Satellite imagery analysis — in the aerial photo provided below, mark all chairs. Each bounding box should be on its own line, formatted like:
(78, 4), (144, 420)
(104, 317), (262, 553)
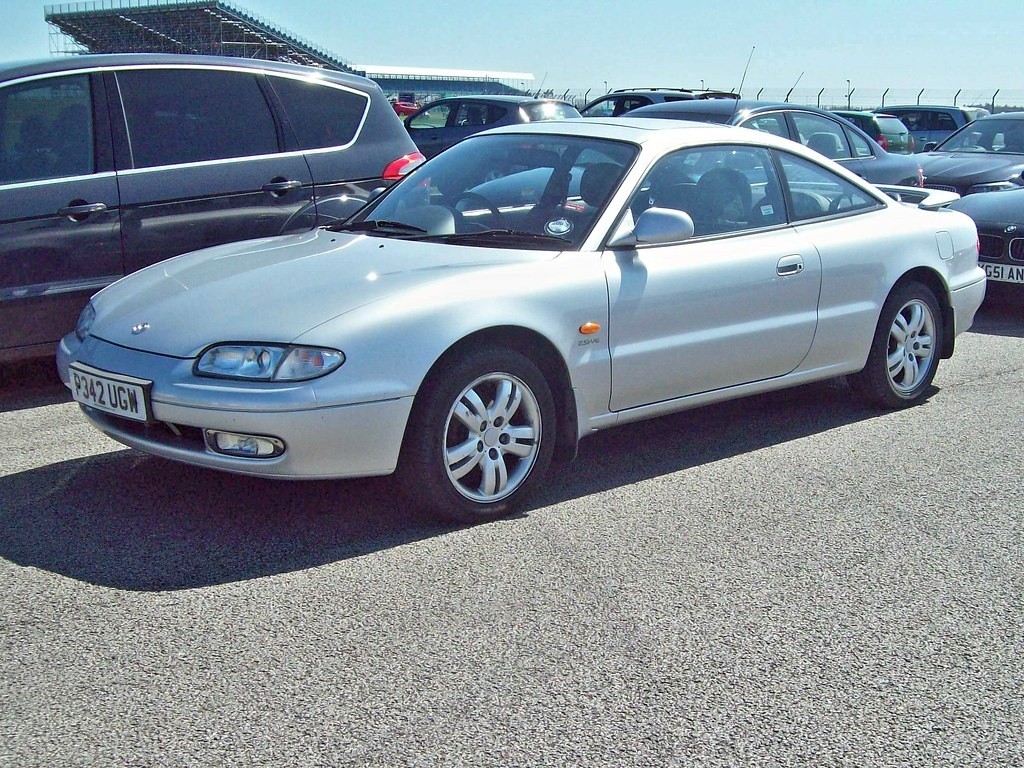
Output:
(997, 125), (1024, 154)
(806, 132), (847, 160)
(518, 162), (833, 257)
(461, 107), (486, 127)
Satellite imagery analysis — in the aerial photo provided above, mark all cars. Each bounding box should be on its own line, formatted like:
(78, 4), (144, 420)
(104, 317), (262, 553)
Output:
(911, 110), (1024, 207)
(455, 100), (925, 241)
(53, 116), (986, 524)
(944, 173), (1024, 302)
(762, 110), (917, 158)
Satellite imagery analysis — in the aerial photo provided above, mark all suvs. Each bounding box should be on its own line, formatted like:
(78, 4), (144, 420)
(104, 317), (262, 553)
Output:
(404, 95), (587, 195)
(394, 102), (429, 119)
(0, 54), (429, 367)
(870, 105), (993, 150)
(577, 87), (758, 130)
